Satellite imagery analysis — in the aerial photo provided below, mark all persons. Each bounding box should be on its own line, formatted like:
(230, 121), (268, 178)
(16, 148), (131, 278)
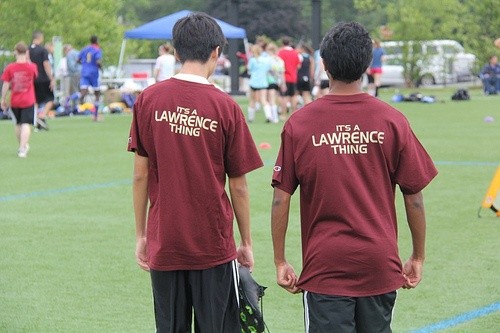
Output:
(127, 15), (265, 333)
(269, 21), (438, 333)
(0, 10), (388, 160)
(480, 55), (500, 94)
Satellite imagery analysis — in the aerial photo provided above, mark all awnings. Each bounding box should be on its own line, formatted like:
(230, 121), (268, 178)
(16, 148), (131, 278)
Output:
(126, 10), (245, 39)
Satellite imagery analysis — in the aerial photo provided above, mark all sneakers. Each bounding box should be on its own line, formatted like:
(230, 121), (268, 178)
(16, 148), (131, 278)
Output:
(238, 262), (270, 333)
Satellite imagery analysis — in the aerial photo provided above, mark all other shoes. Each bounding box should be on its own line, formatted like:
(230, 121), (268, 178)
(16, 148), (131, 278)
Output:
(34, 117), (48, 131)
(18, 143), (29, 156)
(80, 95), (84, 104)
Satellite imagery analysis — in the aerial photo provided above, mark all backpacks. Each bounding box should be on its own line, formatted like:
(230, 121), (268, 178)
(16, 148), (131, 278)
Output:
(452, 88), (471, 99)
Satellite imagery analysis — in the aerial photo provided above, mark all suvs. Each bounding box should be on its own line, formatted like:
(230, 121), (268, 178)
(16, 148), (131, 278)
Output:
(381, 40), (480, 88)
